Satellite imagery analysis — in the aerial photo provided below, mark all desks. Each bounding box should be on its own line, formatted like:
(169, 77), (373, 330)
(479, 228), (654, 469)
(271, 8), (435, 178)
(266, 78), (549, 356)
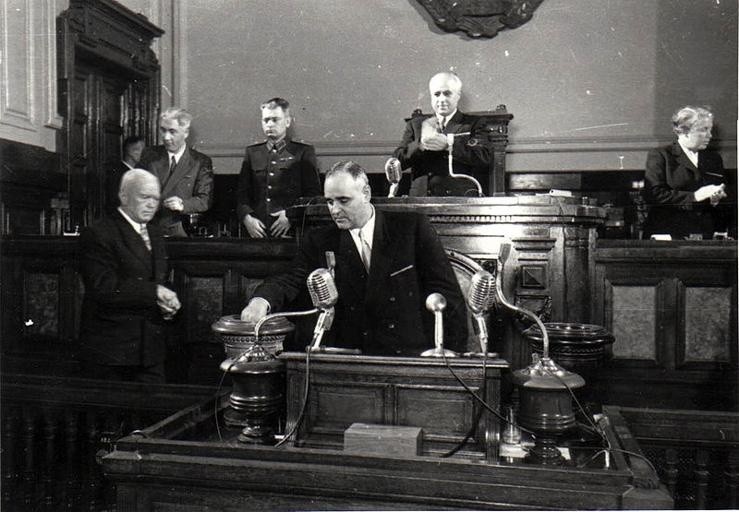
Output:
(2, 198), (735, 409)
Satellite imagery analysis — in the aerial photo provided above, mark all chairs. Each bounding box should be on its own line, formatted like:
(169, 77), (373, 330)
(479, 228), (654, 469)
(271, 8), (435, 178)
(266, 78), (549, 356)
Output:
(404, 102), (515, 195)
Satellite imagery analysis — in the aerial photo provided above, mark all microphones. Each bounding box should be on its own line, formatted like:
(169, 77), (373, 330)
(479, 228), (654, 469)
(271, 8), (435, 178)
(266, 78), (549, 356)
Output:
(425, 293), (447, 317)
(307, 267), (340, 311)
(385, 158), (403, 184)
(467, 272), (496, 314)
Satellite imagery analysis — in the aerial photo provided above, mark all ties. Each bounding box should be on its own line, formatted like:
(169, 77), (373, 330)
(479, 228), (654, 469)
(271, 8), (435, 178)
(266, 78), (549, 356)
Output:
(359, 231), (371, 270)
(170, 156), (177, 172)
(441, 115), (447, 131)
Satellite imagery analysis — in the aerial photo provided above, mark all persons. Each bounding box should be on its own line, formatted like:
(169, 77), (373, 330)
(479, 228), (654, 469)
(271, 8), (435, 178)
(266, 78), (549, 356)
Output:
(391, 70), (495, 195)
(72, 166), (183, 381)
(238, 159), (468, 356)
(235, 97), (323, 239)
(639, 105), (732, 240)
(133, 107), (215, 237)
(105, 135), (146, 216)
